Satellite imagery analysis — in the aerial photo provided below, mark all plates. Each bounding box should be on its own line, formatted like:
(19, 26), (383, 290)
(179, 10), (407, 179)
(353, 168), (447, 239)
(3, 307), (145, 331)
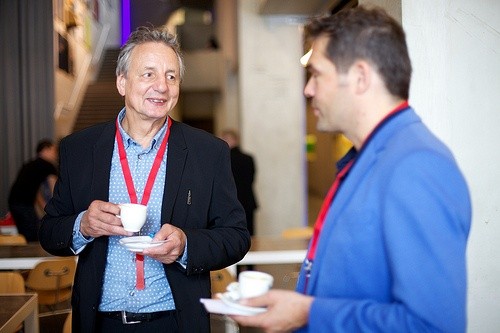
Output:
(222, 293), (266, 312)
(120, 240), (166, 249)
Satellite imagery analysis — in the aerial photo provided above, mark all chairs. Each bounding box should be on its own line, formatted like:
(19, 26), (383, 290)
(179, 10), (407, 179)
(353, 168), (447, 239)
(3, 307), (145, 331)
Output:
(25, 259), (76, 333)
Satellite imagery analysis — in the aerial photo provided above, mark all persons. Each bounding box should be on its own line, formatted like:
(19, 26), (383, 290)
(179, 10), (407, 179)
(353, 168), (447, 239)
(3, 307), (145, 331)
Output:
(38, 22), (252, 333)
(222, 130), (259, 236)
(8, 139), (56, 244)
(228, 7), (472, 333)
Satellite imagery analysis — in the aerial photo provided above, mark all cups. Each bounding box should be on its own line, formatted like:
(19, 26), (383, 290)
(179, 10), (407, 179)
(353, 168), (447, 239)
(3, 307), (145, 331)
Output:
(116, 204), (147, 232)
(239, 271), (273, 296)
